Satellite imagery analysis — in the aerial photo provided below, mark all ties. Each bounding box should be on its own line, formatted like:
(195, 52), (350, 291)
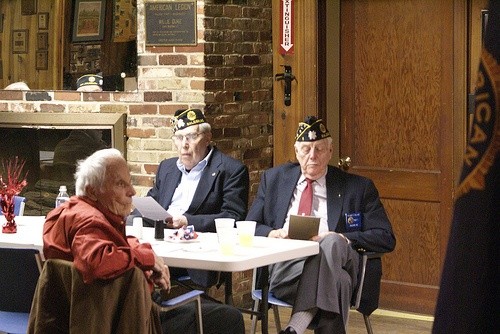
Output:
(298, 178), (316, 216)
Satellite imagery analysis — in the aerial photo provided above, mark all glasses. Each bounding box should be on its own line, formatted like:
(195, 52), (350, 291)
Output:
(172, 130), (207, 142)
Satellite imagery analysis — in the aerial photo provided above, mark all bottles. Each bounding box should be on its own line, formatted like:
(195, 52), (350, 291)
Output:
(55, 186), (69, 208)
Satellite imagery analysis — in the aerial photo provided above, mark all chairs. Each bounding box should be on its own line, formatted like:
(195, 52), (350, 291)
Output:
(0, 247), (46, 334)
(251, 251), (383, 333)
(27, 259), (204, 333)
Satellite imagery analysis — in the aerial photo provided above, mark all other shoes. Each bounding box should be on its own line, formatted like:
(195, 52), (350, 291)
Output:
(279, 326), (297, 334)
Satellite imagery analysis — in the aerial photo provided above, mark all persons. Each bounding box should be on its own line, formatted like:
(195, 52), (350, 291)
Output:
(42, 148), (247, 334)
(123, 109), (250, 287)
(246, 116), (396, 334)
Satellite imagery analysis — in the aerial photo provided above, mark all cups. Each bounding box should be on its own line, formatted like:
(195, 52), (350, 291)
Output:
(155, 220), (164, 239)
(236, 221), (257, 242)
(214, 218), (236, 241)
(133, 217), (143, 238)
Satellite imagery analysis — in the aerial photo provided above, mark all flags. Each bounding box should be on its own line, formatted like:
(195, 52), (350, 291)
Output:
(432, 0), (500, 334)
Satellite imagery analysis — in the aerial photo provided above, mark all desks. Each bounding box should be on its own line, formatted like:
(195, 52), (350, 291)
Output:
(1, 212), (319, 334)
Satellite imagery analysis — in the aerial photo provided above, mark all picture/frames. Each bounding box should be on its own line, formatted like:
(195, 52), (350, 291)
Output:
(72, 0), (107, 43)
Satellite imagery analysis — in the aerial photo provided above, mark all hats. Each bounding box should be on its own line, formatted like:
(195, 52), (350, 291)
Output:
(77, 74), (103, 88)
(295, 115), (331, 142)
(170, 108), (207, 134)
